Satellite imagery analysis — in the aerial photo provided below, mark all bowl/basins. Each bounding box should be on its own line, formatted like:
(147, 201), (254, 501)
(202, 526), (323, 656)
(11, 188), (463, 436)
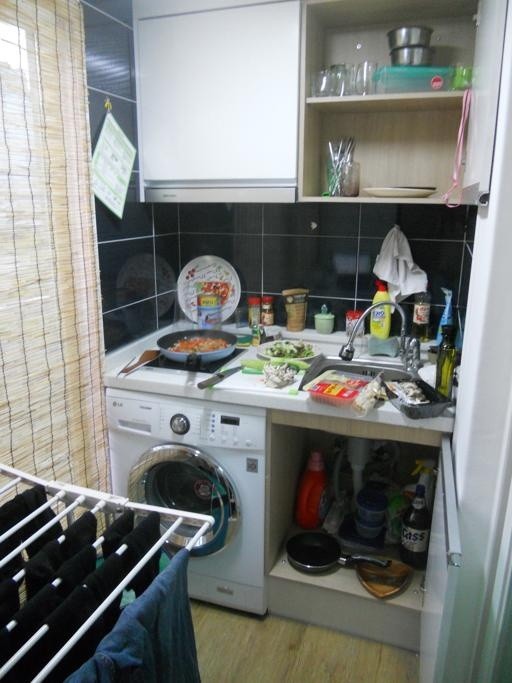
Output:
(386, 22), (435, 50)
(389, 45), (434, 67)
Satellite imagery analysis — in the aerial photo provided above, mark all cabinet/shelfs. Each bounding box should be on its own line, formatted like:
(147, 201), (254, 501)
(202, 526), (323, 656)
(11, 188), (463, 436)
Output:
(131, 1), (302, 190)
(296, 0), (506, 203)
(266, 409), (461, 682)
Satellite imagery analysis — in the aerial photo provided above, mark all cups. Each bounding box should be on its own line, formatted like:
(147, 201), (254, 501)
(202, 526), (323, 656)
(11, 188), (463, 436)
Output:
(313, 61), (379, 97)
(325, 153), (361, 197)
(314, 313), (335, 334)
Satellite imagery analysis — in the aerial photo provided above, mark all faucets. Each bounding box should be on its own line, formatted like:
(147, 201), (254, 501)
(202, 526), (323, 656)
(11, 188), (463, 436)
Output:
(339, 299), (408, 362)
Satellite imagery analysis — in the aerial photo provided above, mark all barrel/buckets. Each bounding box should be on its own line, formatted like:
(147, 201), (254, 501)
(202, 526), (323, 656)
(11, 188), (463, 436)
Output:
(352, 484), (387, 540)
(294, 451), (336, 529)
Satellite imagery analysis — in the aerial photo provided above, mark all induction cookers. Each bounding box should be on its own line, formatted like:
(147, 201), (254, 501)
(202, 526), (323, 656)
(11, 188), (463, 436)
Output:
(143, 346), (248, 374)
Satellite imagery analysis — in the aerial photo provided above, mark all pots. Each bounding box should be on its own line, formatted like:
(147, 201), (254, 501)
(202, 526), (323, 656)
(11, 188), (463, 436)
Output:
(285, 531), (388, 574)
(155, 327), (238, 369)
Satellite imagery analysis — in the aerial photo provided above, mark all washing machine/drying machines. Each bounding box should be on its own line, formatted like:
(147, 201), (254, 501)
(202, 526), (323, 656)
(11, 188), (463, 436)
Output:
(105, 388), (268, 618)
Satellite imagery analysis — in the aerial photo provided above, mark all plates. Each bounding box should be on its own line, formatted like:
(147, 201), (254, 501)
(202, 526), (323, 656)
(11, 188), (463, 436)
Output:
(256, 339), (323, 361)
(362, 187), (438, 198)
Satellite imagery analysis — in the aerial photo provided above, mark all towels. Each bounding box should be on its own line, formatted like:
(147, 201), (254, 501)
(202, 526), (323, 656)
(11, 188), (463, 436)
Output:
(372, 226), (428, 314)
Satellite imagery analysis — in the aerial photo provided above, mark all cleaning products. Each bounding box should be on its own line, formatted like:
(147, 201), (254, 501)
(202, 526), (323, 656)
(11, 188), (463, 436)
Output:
(369, 279), (392, 340)
(410, 458), (438, 515)
(385, 489), (410, 543)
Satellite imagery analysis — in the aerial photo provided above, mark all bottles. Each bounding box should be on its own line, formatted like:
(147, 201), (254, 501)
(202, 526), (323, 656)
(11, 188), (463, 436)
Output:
(399, 483), (432, 571)
(435, 324), (458, 400)
(248, 296), (261, 328)
(261, 295), (275, 326)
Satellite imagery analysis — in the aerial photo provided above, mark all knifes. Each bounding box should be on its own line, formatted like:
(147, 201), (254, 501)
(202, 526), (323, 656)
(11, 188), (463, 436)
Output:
(198, 366), (242, 389)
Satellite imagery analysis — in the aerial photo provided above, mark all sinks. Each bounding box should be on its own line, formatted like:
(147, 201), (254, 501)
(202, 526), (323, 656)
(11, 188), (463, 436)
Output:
(297, 352), (422, 393)
